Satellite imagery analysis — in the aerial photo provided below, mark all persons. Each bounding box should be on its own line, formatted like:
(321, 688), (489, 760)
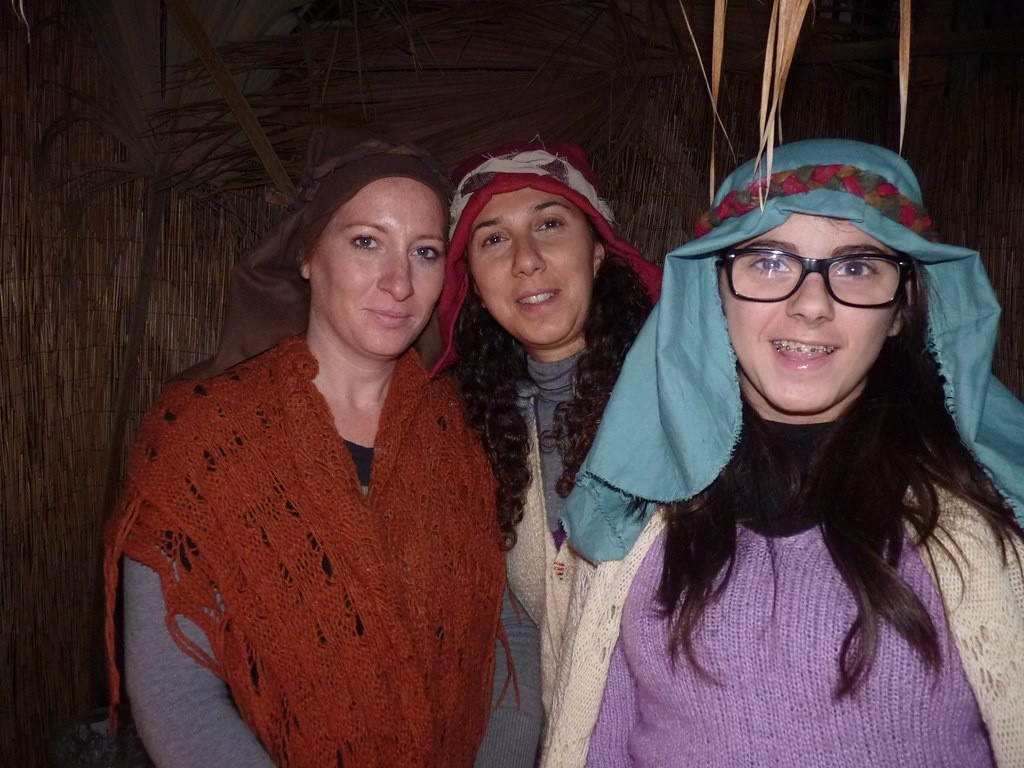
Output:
(535, 141), (1024, 768)
(433, 139), (666, 627)
(102, 138), (506, 768)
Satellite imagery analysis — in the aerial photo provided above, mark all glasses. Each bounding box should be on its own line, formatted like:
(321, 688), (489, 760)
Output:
(715, 246), (915, 308)
